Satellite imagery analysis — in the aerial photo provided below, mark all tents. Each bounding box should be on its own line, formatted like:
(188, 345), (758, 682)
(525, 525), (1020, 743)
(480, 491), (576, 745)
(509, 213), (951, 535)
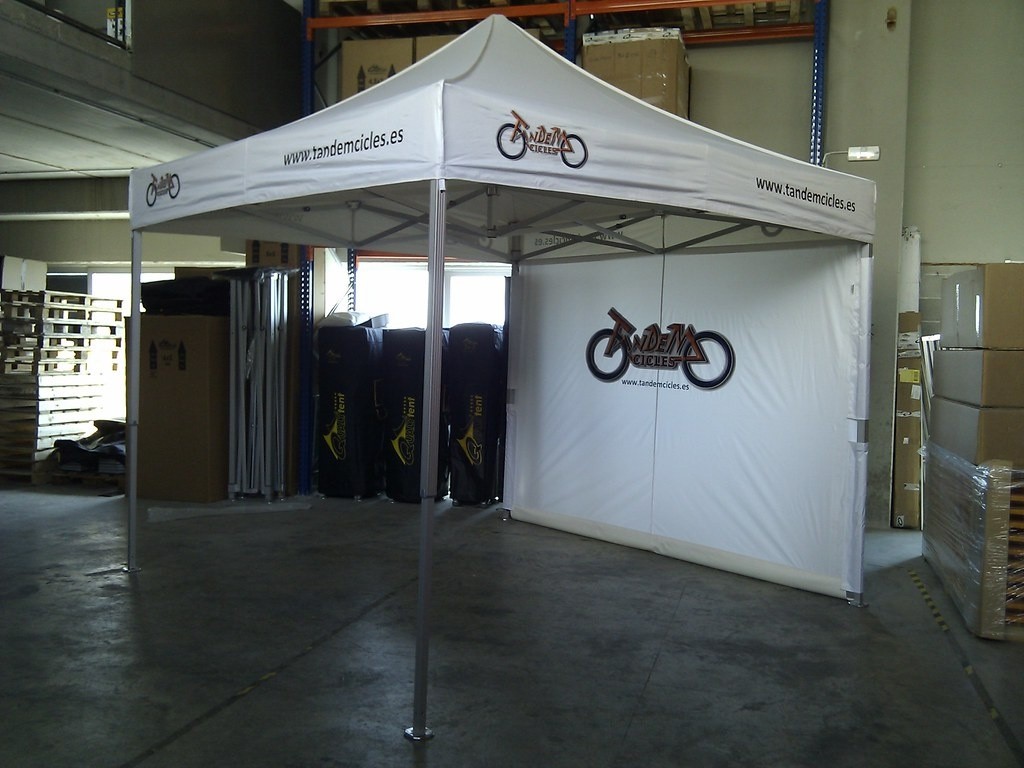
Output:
(125, 15), (878, 743)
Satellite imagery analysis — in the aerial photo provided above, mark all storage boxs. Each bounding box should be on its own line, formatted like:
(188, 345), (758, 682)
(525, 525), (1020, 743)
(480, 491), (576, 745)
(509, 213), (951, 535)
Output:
(582, 27), (690, 120)
(124, 313), (227, 504)
(928, 262), (1024, 471)
